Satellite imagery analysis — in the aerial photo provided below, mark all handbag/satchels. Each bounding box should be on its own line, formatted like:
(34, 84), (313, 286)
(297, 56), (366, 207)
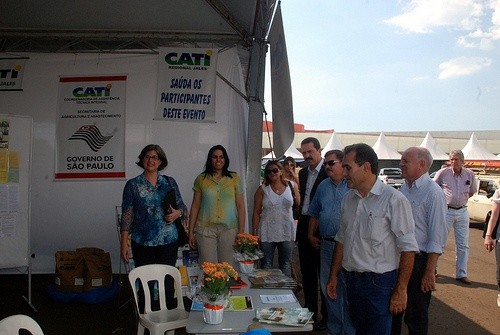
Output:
(162, 175), (189, 248)
(481, 210), (500, 239)
(288, 181), (299, 221)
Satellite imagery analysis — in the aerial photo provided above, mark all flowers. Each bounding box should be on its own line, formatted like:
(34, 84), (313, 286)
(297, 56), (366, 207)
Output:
(233, 233), (263, 261)
(202, 262), (238, 306)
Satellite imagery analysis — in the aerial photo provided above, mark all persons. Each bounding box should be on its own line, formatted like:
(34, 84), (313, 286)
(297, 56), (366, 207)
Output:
(295, 137), (329, 329)
(189, 144), (246, 268)
(484, 188), (500, 307)
(121, 144), (188, 313)
(253, 161), (300, 277)
(283, 157), (300, 189)
(479, 181), (487, 193)
(326, 142), (420, 334)
(433, 150), (477, 286)
(397, 147), (448, 335)
(306, 150), (355, 335)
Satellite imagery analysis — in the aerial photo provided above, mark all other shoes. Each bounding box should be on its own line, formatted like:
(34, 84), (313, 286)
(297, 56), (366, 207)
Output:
(456, 277), (471, 284)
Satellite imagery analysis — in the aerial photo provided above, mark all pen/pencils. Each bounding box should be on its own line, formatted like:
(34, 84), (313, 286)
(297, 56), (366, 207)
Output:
(266, 296), (270, 301)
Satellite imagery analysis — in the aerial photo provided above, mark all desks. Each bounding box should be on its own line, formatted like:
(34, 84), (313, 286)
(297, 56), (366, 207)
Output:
(186, 267), (313, 332)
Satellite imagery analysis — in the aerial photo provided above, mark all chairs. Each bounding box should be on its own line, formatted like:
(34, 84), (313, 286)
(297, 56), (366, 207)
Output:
(128, 264), (189, 335)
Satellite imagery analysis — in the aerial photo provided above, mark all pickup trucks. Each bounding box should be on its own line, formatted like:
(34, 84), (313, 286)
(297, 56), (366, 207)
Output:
(378, 168), (406, 188)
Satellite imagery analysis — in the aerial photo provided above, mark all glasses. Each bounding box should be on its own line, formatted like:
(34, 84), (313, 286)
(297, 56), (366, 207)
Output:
(285, 162), (294, 167)
(212, 155), (224, 159)
(266, 168), (279, 174)
(322, 160), (340, 167)
(143, 155), (159, 160)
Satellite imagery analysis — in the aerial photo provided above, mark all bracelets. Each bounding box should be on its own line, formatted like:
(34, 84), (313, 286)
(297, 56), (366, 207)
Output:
(486, 235), (491, 236)
(179, 214), (182, 218)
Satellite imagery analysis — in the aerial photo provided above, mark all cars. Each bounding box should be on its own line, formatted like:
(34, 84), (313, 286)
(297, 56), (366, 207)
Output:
(430, 172), (437, 180)
(468, 168), (486, 175)
(466, 175), (500, 224)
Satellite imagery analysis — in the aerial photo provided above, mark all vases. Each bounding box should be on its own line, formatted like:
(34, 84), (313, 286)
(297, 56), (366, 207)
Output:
(203, 304), (223, 324)
(240, 262), (254, 273)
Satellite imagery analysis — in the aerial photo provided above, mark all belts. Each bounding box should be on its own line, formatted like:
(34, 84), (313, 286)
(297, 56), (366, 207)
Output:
(321, 236), (335, 241)
(448, 205), (465, 209)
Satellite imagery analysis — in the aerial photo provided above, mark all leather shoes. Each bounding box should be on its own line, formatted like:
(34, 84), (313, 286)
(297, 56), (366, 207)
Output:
(313, 317), (328, 330)
